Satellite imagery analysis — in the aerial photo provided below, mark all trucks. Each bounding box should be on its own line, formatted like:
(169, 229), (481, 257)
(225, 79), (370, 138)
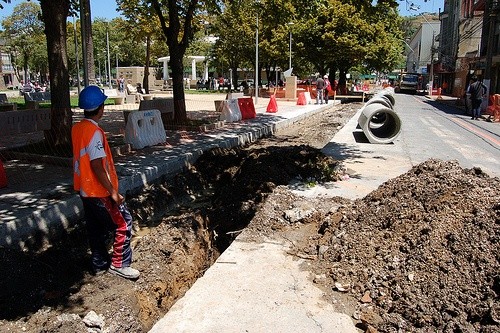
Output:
(393, 73), (420, 95)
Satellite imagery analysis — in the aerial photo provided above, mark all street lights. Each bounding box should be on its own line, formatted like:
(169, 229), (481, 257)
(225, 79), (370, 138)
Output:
(287, 21), (295, 69)
(114, 45), (119, 79)
(102, 19), (112, 90)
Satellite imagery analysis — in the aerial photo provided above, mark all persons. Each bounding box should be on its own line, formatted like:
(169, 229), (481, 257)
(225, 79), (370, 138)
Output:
(71, 85), (140, 279)
(16, 78), (51, 94)
(116, 75), (126, 93)
(469, 75), (487, 121)
(316, 74), (331, 105)
(184, 75), (313, 92)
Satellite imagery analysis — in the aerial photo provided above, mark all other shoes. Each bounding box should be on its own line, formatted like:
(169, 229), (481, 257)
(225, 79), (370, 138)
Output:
(476, 118), (478, 120)
(471, 118), (474, 120)
(109, 266), (140, 279)
(93, 256), (107, 267)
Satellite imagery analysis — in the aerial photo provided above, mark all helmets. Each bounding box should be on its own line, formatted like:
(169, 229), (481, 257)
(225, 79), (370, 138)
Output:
(78, 85), (108, 109)
(324, 75), (328, 78)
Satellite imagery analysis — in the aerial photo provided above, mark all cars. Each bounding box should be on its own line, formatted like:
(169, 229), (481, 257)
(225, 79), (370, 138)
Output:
(215, 78), (255, 88)
(196, 79), (210, 91)
(20, 84), (32, 96)
(265, 74), (317, 87)
